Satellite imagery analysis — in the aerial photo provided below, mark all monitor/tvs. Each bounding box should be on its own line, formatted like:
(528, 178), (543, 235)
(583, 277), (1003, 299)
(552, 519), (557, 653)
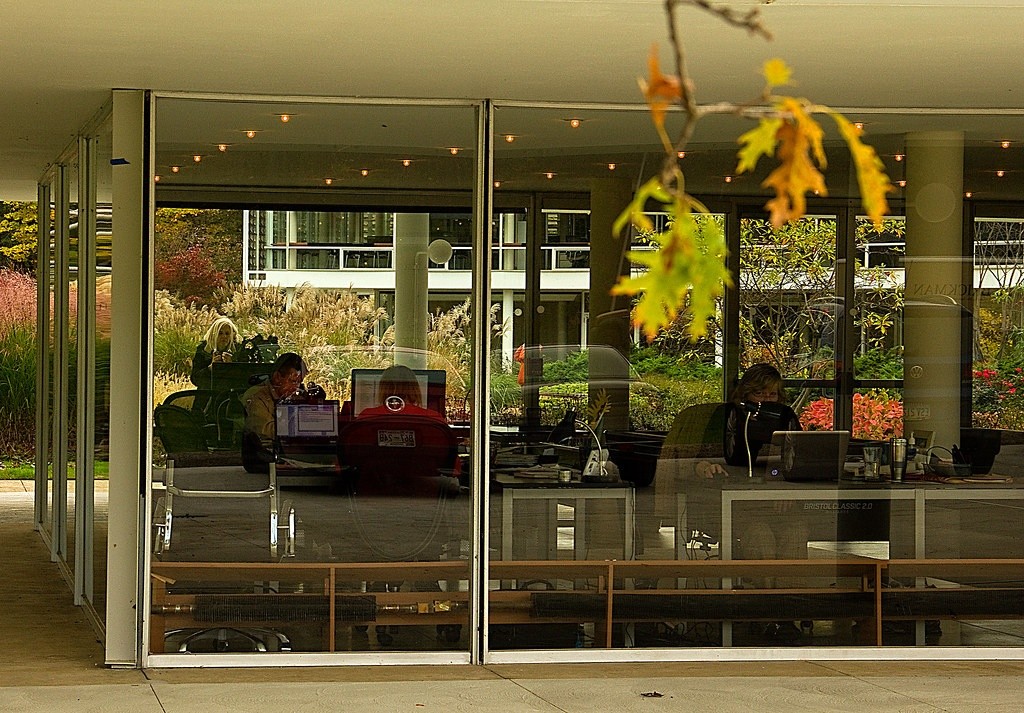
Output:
(352, 368), (446, 420)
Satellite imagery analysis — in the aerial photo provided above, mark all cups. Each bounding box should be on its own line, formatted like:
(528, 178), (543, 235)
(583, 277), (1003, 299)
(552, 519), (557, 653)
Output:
(863, 447), (882, 481)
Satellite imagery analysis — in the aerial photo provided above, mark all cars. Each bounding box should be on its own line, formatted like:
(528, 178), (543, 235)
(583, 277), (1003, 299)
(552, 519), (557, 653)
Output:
(481, 344), (667, 491)
(153, 338), (468, 498)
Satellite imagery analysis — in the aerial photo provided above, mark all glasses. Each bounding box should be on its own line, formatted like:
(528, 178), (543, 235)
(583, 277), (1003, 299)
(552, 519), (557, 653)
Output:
(752, 391), (778, 398)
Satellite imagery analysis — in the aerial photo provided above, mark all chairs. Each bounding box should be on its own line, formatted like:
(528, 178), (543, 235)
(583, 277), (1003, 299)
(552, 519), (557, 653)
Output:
(153, 404), (215, 467)
(653, 403), (722, 593)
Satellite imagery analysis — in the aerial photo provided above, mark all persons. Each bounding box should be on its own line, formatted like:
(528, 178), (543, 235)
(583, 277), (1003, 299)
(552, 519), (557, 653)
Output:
(191, 318), (240, 446)
(232, 352), (310, 473)
(356, 363), (462, 478)
(695, 365), (809, 588)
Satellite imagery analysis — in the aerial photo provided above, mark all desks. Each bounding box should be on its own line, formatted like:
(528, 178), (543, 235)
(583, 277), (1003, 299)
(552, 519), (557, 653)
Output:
(488, 478), (640, 649)
(276, 466), (469, 522)
(671, 476), (1024, 647)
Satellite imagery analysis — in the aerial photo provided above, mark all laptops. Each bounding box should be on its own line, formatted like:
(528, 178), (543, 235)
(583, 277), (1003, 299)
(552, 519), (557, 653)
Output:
(764, 430), (849, 482)
(274, 400), (340, 455)
